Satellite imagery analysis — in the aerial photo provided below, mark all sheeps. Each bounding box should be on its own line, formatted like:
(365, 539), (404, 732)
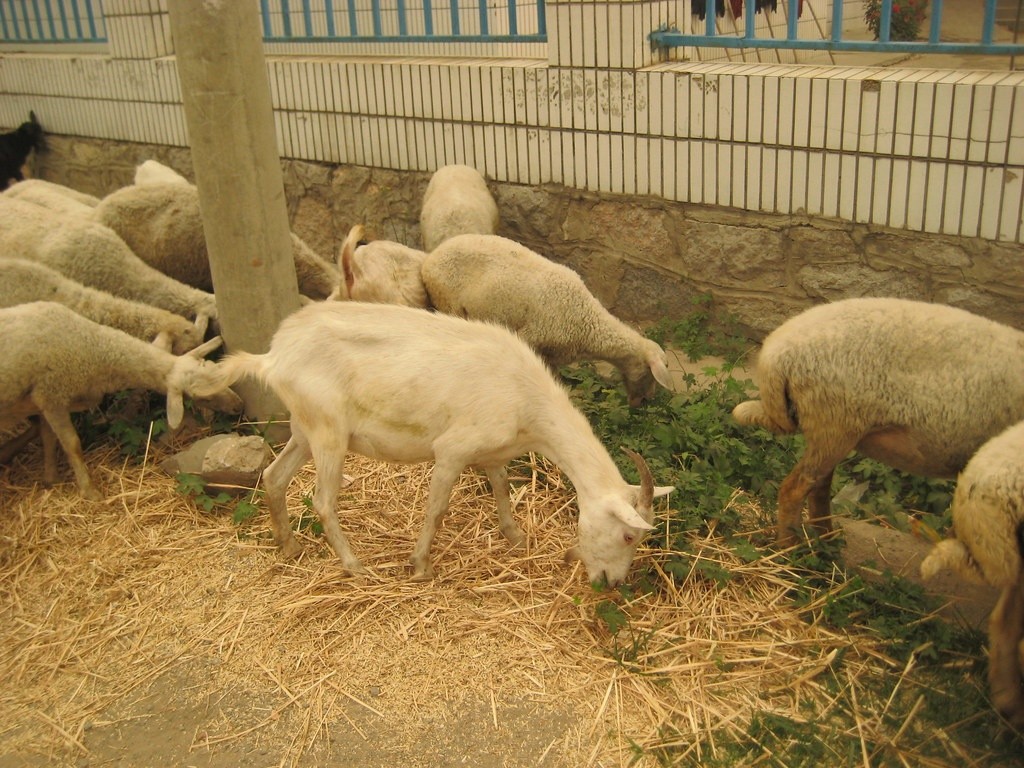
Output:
(731, 297), (1022, 711)
(334, 163), (667, 398)
(0, 114), (339, 485)
(221, 302), (674, 591)
